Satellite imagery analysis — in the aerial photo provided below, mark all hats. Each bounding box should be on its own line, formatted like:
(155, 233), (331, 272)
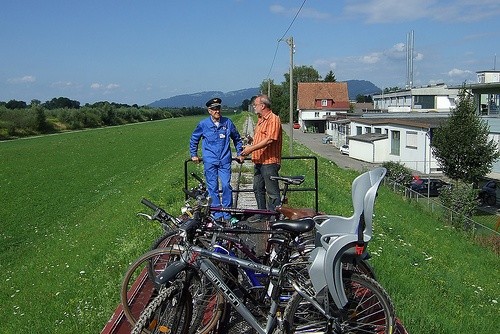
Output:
(205, 98), (222, 110)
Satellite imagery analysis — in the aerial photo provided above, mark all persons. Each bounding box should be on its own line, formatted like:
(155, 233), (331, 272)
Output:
(240, 95), (283, 221)
(190, 98), (245, 222)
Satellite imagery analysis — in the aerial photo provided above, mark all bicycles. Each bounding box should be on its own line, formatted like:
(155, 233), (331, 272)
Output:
(477, 188), (497, 207)
(121, 169), (397, 334)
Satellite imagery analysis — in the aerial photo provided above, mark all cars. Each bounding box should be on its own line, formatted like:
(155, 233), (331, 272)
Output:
(322, 135), (333, 144)
(406, 177), (455, 198)
(476, 180), (500, 197)
(339, 144), (349, 154)
(294, 123), (300, 129)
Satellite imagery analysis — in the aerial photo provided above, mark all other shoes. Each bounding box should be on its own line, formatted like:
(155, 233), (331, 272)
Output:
(248, 215), (262, 222)
(224, 219), (235, 226)
(264, 218), (279, 224)
(216, 216), (224, 223)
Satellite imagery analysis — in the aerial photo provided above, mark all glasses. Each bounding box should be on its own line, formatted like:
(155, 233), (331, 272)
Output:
(253, 102), (264, 106)
